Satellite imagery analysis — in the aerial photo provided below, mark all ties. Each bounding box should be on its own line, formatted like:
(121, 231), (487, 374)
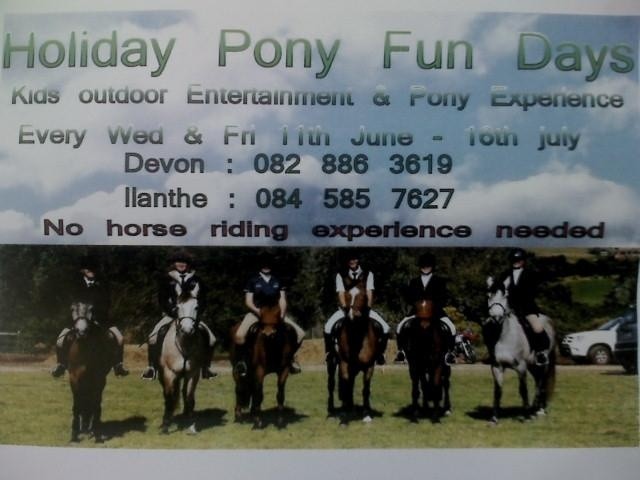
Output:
(352, 273), (358, 280)
(180, 274), (186, 286)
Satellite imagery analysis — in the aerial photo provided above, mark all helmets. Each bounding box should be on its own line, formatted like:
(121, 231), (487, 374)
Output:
(173, 253), (189, 265)
(507, 248), (527, 263)
(418, 252), (434, 269)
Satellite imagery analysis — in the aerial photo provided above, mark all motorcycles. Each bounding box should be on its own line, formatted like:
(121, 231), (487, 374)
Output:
(453, 328), (478, 363)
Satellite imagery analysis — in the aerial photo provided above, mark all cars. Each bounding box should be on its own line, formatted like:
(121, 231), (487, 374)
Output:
(560, 316), (637, 374)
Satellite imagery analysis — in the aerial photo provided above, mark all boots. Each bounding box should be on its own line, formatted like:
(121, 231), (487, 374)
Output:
(114, 344), (129, 376)
(376, 332), (389, 365)
(289, 342), (302, 374)
(202, 345), (218, 379)
(143, 343), (157, 378)
(394, 332), (406, 362)
(52, 346), (66, 378)
(447, 334), (457, 364)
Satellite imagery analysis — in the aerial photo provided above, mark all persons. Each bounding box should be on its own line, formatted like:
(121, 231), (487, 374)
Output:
(49, 255), (130, 380)
(393, 252), (477, 365)
(323, 248), (391, 369)
(481, 248), (551, 368)
(140, 251), (220, 381)
(234, 250), (307, 378)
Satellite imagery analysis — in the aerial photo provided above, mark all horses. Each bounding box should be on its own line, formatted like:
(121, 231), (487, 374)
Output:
(482, 274), (558, 428)
(228, 285), (298, 433)
(66, 291), (116, 446)
(405, 294), (458, 427)
(157, 281), (214, 435)
(326, 271), (383, 427)
